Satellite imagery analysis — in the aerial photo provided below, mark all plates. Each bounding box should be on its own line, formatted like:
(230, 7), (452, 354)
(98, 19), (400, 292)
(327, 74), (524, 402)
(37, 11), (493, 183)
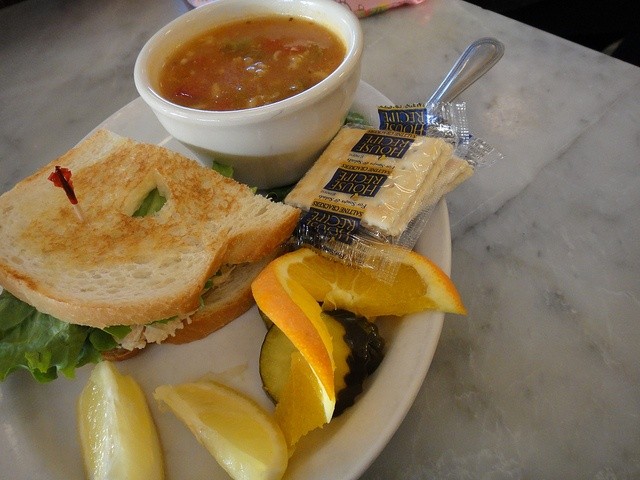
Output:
(0, 82), (452, 479)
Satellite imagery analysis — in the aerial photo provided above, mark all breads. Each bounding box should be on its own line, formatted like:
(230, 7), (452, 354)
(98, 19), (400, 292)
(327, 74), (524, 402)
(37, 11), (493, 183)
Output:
(0, 126), (301, 358)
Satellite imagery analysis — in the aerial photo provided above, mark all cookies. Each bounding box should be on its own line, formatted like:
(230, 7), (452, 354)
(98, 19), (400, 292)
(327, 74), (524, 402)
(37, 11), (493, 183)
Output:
(284, 126), (454, 239)
(436, 159), (479, 190)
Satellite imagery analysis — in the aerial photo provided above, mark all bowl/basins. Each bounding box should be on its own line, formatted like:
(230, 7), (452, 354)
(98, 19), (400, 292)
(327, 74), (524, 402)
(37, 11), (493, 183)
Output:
(134, 0), (365, 189)
